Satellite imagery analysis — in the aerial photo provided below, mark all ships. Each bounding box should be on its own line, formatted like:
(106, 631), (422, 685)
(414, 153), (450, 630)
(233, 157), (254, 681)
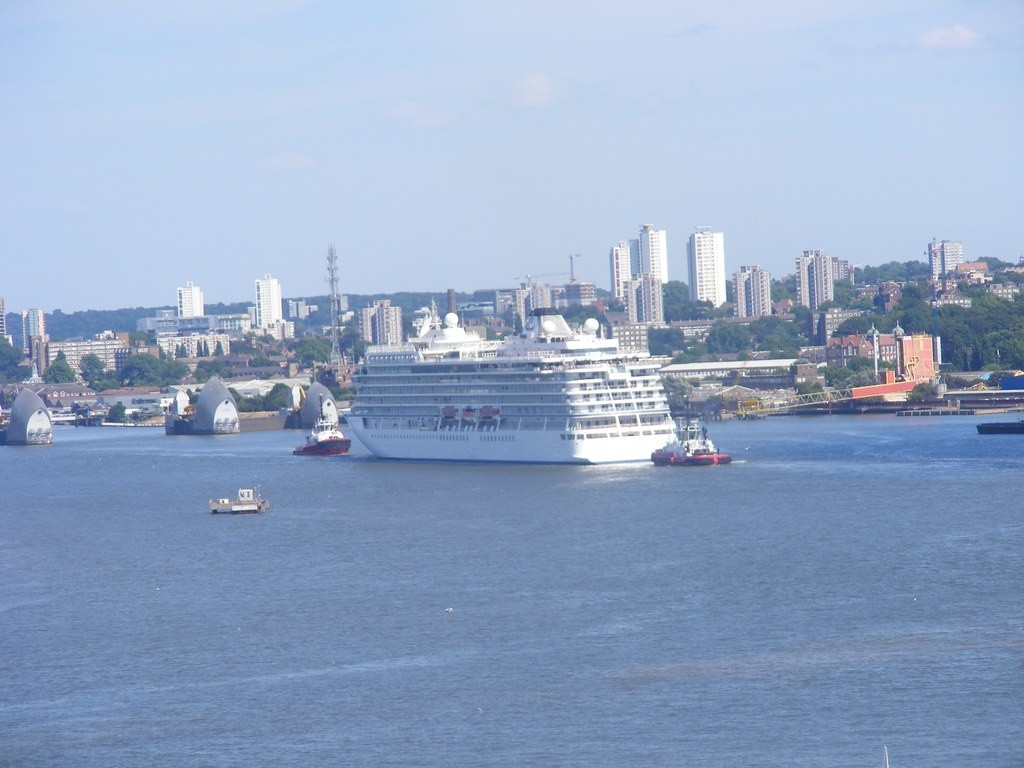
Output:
(341, 312), (680, 465)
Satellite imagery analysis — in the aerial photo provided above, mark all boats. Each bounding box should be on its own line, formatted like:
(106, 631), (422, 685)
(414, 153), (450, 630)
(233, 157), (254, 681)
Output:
(650, 418), (733, 466)
(479, 405), (500, 419)
(462, 405), (478, 419)
(441, 405), (460, 419)
(208, 484), (270, 514)
(292, 392), (351, 455)
(976, 419), (1024, 434)
